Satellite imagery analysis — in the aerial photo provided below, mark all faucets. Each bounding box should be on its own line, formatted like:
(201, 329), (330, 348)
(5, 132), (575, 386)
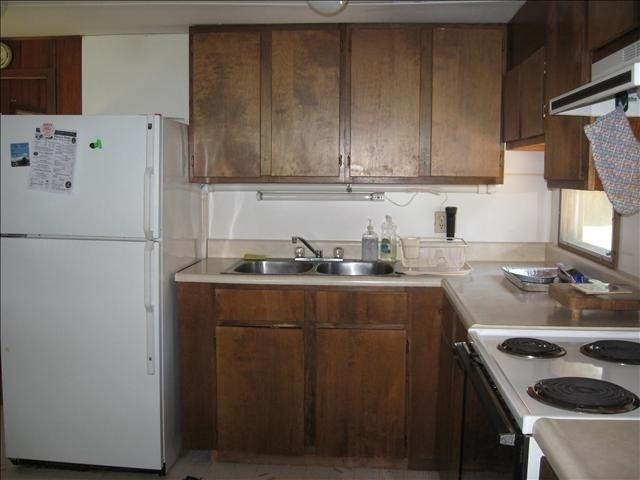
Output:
(292, 236), (322, 258)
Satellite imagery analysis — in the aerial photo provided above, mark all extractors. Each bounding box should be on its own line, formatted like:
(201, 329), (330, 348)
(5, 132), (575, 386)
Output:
(548, 38), (640, 120)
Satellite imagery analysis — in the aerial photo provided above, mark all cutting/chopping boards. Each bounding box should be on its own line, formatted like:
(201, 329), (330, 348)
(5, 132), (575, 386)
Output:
(548, 277), (640, 313)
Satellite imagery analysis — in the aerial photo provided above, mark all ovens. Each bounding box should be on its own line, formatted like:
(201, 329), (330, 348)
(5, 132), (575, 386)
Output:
(455, 339), (531, 480)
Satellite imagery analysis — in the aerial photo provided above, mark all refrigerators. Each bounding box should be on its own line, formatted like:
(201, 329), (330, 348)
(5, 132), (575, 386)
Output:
(0, 112), (208, 477)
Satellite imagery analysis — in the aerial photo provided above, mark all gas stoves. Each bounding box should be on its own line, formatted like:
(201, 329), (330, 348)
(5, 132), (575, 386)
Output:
(468, 326), (640, 439)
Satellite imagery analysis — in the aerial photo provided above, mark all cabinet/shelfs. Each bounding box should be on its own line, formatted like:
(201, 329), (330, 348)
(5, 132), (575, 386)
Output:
(442, 287), (524, 480)
(544, 0), (588, 190)
(2, 241), (197, 476)
(346, 25), (503, 185)
(188, 24), (345, 184)
(503, 0), (544, 151)
(177, 284), (441, 465)
(1, 113), (200, 240)
(588, 0), (640, 63)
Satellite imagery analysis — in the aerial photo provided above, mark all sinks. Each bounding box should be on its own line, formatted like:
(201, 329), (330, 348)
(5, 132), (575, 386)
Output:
(220, 256), (312, 276)
(315, 259), (400, 277)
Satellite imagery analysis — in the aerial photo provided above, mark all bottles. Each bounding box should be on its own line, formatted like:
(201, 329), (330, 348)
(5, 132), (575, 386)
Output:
(380, 215), (398, 264)
